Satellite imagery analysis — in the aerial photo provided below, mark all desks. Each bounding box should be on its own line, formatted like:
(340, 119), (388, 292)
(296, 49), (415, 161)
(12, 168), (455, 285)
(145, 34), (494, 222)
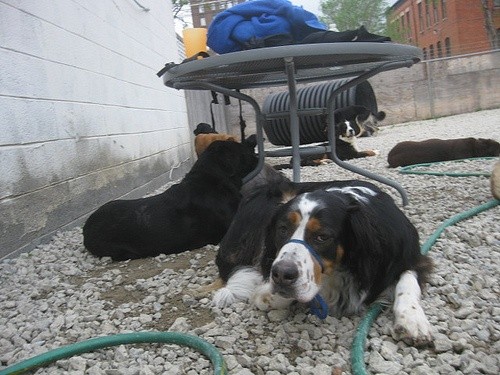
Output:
(162, 42), (425, 209)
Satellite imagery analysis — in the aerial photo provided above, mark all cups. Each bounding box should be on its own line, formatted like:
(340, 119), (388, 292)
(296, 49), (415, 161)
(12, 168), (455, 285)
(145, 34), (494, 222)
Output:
(182, 27), (207, 62)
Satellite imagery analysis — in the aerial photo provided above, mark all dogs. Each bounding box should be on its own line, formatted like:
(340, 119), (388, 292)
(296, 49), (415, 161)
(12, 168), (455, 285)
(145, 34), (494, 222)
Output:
(387, 138), (500, 168)
(191, 181), (436, 348)
(322, 106), (385, 139)
(82, 139), (256, 263)
(272, 139), (376, 169)
